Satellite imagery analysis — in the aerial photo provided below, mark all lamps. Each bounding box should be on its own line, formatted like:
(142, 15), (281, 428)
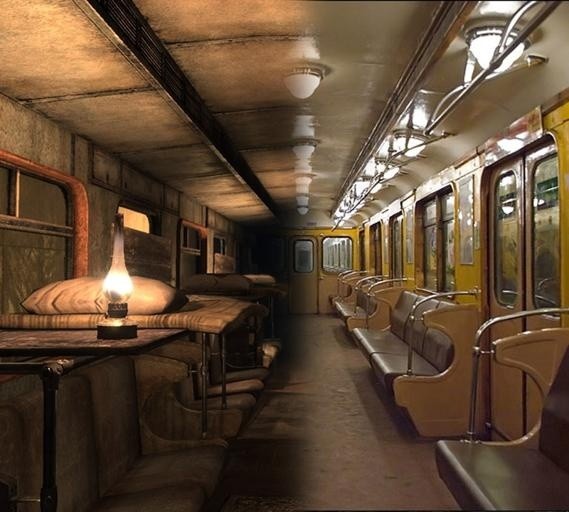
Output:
(280, 58), (328, 217)
(467, 28), (530, 74)
(333, 136), (408, 230)
(98, 212), (138, 340)
(396, 135), (425, 157)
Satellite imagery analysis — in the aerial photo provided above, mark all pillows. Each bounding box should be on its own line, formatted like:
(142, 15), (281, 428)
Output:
(18, 273), (188, 314)
(184, 270), (276, 295)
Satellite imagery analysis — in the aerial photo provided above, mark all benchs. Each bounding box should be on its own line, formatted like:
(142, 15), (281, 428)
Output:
(437, 308), (568, 509)
(330, 271), (414, 338)
(0, 321), (283, 512)
(348, 278), (481, 441)
